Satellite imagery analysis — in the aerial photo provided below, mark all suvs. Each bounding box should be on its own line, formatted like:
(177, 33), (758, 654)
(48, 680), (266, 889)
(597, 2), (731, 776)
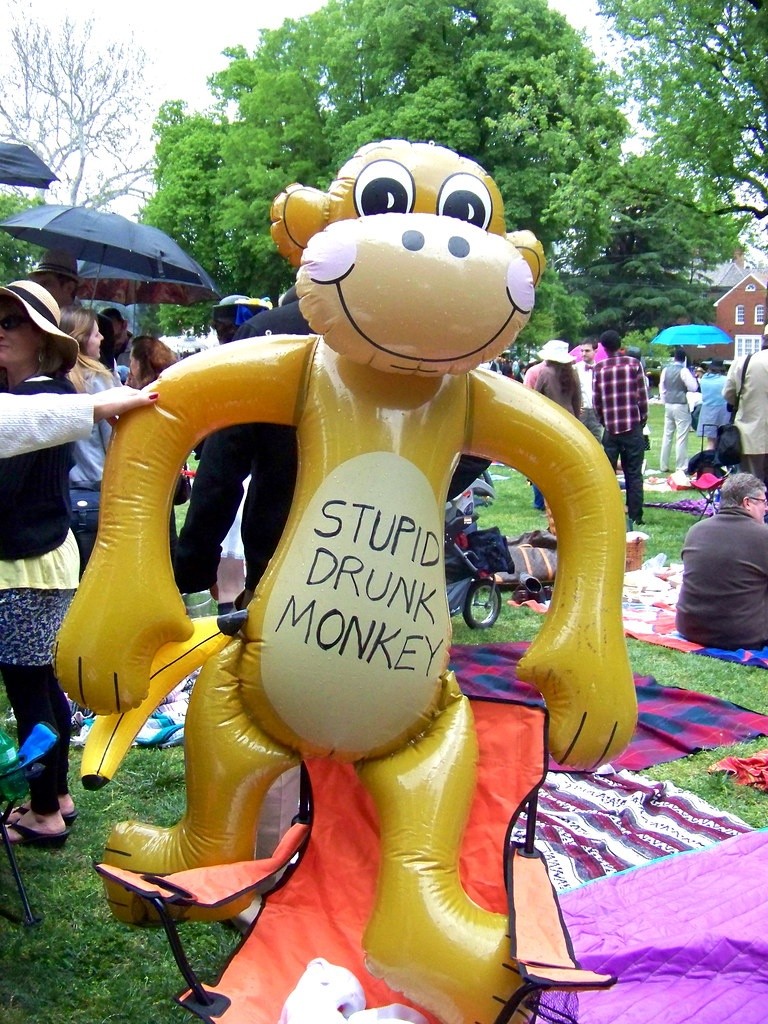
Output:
(642, 356), (675, 386)
(158, 336), (208, 362)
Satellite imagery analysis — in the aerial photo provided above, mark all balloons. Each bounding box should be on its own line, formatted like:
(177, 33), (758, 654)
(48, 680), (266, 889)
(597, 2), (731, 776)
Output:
(53, 142), (638, 1024)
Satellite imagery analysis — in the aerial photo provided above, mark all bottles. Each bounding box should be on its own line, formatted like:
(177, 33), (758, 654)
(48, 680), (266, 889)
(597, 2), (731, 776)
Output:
(0, 726), (30, 803)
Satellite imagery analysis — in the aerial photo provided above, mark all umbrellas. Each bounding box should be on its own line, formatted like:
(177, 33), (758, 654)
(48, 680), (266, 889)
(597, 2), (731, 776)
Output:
(77, 260), (221, 335)
(0, 205), (203, 308)
(0, 142), (62, 189)
(651, 325), (733, 345)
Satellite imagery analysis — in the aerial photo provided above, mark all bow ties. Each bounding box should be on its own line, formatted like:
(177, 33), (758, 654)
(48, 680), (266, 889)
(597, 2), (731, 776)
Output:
(585, 364), (594, 371)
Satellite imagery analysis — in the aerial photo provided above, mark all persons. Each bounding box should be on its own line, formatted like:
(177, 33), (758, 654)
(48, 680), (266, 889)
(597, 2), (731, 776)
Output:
(658, 350), (729, 474)
(0, 280), (490, 890)
(675, 474), (768, 649)
(488, 330), (649, 525)
(722, 349), (768, 480)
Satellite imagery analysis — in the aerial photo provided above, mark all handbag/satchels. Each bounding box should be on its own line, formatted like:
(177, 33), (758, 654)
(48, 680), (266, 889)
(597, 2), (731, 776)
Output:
(175, 473), (192, 506)
(67, 489), (100, 533)
(713, 423), (742, 464)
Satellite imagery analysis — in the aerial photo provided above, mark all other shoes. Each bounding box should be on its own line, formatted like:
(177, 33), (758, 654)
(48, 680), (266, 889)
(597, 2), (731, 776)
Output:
(513, 575), (541, 601)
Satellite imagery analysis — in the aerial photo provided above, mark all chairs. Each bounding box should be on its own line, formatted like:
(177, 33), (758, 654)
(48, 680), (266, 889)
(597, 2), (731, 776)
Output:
(690, 424), (728, 521)
(93, 693), (617, 1024)
(1, 721), (58, 928)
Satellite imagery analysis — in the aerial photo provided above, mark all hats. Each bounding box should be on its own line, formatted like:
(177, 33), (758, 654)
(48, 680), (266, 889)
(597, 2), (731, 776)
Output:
(0, 280), (79, 370)
(538, 340), (577, 363)
(28, 251), (82, 285)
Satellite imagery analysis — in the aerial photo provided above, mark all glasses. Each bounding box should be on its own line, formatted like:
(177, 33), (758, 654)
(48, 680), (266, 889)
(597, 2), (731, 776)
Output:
(738, 495), (768, 507)
(0, 314), (29, 331)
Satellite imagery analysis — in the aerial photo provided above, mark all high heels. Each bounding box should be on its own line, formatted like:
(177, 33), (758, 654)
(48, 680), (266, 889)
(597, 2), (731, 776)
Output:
(0, 817), (71, 848)
(0, 802), (78, 827)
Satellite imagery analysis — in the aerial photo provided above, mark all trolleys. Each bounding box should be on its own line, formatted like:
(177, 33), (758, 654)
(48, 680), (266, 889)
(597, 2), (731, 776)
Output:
(443, 514), (560, 629)
